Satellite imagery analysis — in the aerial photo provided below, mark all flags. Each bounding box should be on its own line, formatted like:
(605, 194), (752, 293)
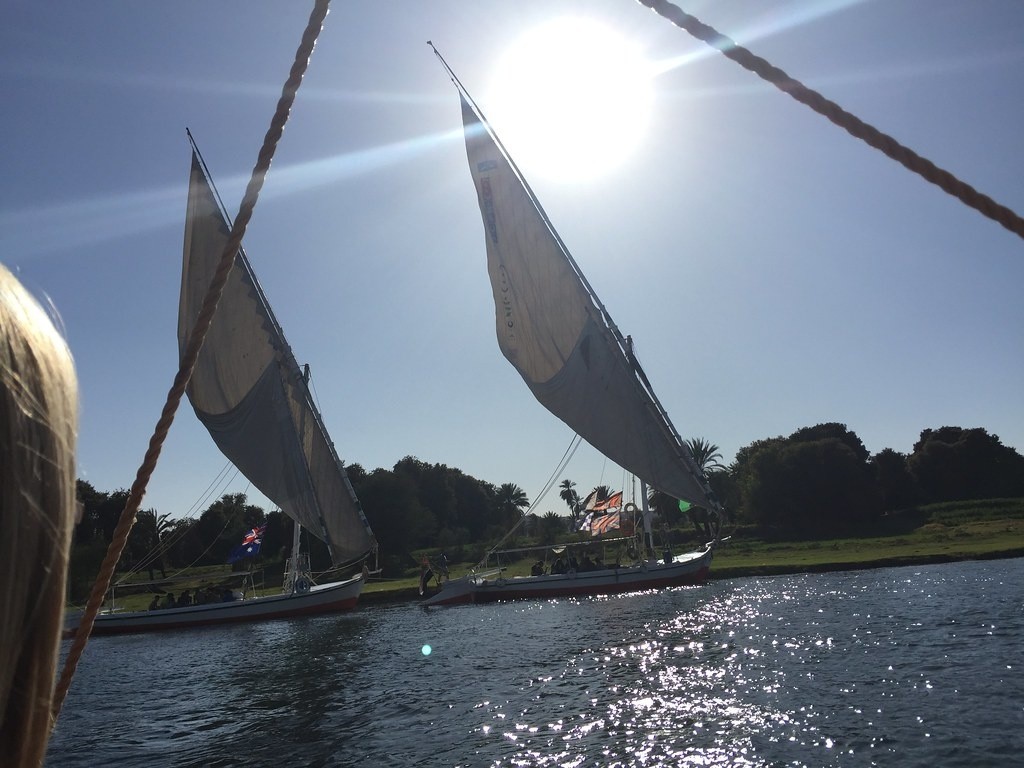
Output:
(579, 512), (595, 532)
(226, 519), (267, 564)
(591, 510), (620, 536)
(580, 491), (598, 511)
(593, 492), (621, 510)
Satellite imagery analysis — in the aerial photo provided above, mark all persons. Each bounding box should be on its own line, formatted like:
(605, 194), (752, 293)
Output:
(529, 561), (549, 575)
(435, 552), (450, 582)
(146, 582), (236, 610)
(594, 557), (608, 570)
(549, 555), (578, 574)
(420, 553), (429, 582)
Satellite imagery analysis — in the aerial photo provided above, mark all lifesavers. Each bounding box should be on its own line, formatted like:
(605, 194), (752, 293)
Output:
(493, 577), (507, 588)
(620, 519), (635, 537)
(641, 565), (649, 575)
(566, 569), (577, 579)
(625, 502), (639, 517)
(627, 548), (639, 559)
(296, 577), (311, 593)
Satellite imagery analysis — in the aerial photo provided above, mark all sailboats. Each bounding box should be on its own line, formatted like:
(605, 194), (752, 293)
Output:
(62, 127), (381, 638)
(418, 41), (732, 606)
